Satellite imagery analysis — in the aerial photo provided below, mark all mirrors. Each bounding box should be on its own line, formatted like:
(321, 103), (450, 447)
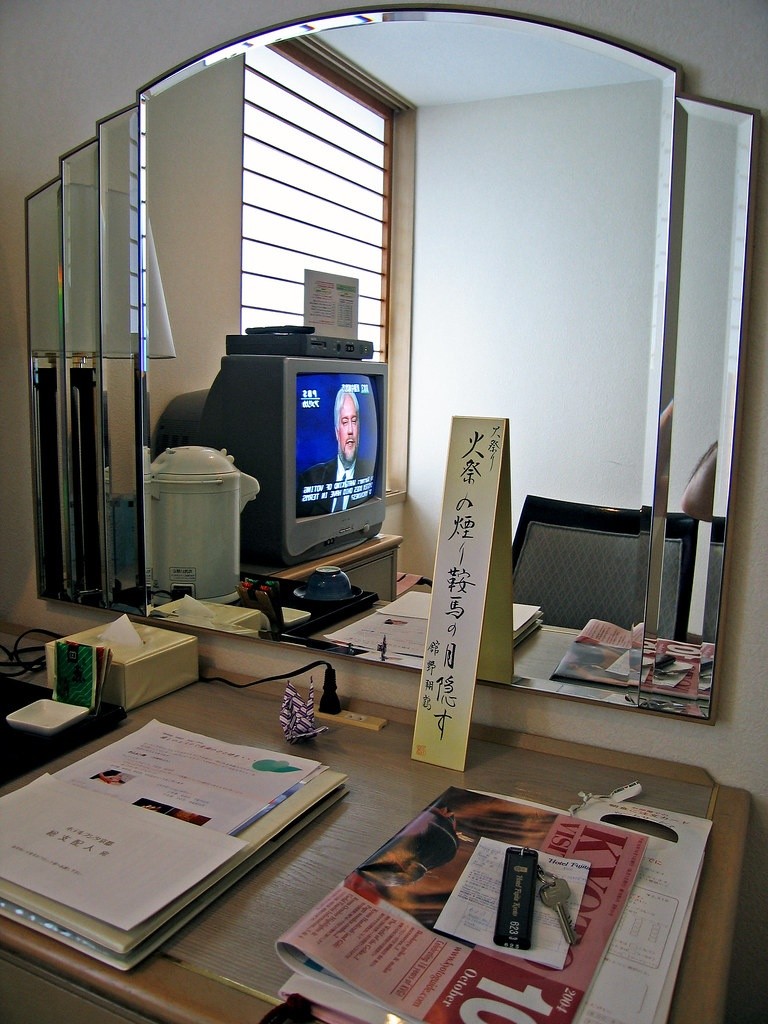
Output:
(24, 3), (757, 729)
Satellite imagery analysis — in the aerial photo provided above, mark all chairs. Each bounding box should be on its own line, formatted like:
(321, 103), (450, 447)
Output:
(510, 493), (725, 646)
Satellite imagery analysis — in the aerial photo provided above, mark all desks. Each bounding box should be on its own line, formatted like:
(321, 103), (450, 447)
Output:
(0, 628), (752, 1024)
(243, 535), (402, 605)
(299, 596), (716, 721)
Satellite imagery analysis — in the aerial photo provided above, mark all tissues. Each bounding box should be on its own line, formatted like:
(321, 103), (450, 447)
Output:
(44, 613), (200, 713)
(151, 593), (262, 633)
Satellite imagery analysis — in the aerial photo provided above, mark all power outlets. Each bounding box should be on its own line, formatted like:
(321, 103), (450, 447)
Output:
(314, 703), (387, 732)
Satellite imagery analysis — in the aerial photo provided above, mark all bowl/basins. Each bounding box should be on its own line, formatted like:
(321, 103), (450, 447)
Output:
(306, 566), (352, 599)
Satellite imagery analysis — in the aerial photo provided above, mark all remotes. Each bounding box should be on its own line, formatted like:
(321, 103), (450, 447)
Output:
(245, 325), (315, 334)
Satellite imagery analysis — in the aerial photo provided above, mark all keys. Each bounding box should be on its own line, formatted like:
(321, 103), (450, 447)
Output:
(654, 670), (679, 679)
(540, 879), (575, 945)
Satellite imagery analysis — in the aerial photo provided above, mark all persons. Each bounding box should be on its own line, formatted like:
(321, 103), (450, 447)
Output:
(654, 400), (673, 519)
(682, 442), (718, 523)
(297, 388), (376, 516)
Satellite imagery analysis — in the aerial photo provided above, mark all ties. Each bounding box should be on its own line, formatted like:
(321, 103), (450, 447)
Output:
(333, 473), (346, 512)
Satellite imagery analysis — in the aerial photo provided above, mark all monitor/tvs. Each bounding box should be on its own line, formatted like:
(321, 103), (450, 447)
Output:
(155, 354), (388, 566)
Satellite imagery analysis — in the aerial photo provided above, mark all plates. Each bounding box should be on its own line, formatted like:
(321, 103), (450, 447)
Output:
(293, 584), (363, 603)
(6, 699), (91, 736)
(282, 607), (311, 627)
